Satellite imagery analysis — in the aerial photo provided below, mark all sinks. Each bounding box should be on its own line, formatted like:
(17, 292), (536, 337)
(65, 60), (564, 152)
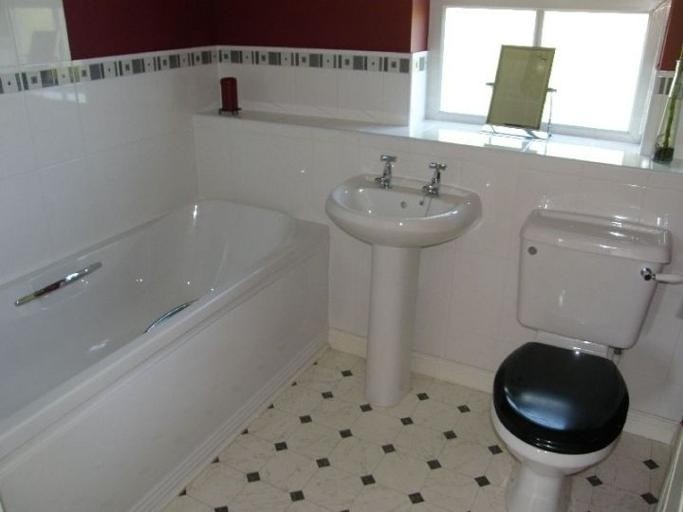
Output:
(327, 174), (484, 247)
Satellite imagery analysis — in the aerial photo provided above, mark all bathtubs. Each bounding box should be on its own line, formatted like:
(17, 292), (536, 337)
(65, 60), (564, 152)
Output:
(0, 197), (327, 461)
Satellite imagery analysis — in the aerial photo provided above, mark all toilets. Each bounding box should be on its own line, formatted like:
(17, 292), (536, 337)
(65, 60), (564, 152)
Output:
(490, 209), (671, 511)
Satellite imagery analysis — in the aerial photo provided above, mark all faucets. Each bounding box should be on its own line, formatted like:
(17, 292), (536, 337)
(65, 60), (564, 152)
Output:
(422, 162), (447, 192)
(374, 155), (395, 188)
